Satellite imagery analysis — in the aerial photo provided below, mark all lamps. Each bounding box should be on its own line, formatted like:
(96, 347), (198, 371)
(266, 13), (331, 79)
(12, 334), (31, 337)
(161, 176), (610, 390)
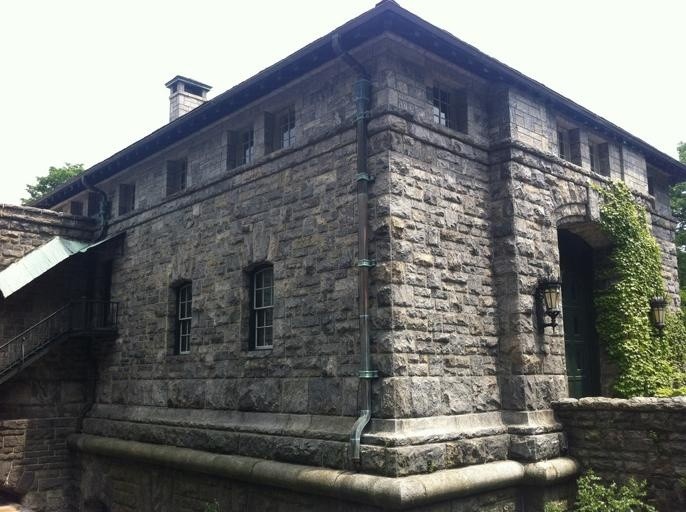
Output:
(539, 263), (561, 334)
(649, 293), (667, 340)
